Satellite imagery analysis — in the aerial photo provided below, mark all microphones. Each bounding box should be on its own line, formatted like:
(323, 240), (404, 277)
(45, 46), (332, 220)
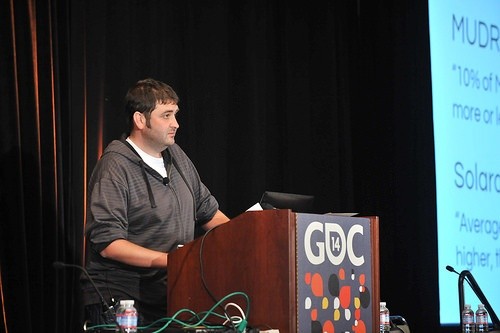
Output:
(53, 262), (127, 333)
(163, 178), (169, 184)
(446, 266), (498, 329)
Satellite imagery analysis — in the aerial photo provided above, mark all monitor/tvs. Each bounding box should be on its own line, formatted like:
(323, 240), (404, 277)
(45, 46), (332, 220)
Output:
(259, 191), (314, 214)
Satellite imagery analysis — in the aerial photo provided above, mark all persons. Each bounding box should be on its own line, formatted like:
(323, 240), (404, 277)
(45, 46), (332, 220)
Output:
(84, 78), (230, 333)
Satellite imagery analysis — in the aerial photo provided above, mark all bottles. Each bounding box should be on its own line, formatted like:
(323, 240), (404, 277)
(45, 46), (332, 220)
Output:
(462, 305), (473, 333)
(477, 304), (489, 332)
(115, 298), (136, 333)
(379, 301), (389, 332)
